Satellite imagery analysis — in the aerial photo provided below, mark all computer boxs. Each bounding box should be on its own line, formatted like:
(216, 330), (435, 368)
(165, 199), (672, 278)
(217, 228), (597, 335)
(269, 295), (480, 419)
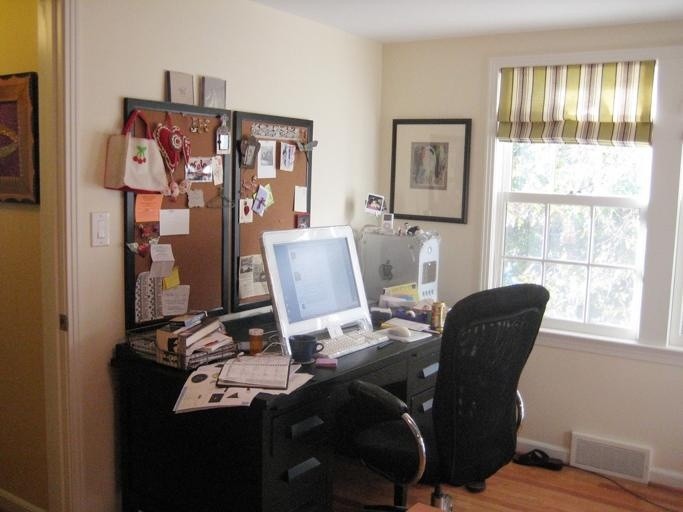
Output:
(359, 225), (438, 302)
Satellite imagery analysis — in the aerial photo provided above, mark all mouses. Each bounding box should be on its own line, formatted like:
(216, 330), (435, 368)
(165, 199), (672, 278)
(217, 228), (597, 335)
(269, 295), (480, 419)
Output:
(388, 327), (410, 338)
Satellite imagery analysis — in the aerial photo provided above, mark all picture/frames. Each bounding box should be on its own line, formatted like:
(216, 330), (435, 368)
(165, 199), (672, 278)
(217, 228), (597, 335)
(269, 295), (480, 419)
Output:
(389, 118), (472, 225)
(202, 76), (227, 110)
(122, 96), (229, 337)
(168, 70), (194, 105)
(231, 110), (313, 313)
(0, 71), (42, 204)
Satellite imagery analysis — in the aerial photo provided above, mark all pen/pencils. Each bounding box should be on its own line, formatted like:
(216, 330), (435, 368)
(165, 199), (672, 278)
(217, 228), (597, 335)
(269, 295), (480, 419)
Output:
(377, 340), (393, 349)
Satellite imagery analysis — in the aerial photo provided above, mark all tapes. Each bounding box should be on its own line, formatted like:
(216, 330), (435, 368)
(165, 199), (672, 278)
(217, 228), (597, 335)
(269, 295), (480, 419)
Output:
(406, 311), (416, 318)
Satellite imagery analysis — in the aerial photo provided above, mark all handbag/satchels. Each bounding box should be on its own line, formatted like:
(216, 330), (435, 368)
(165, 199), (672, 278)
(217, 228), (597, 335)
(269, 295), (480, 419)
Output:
(104, 110), (168, 194)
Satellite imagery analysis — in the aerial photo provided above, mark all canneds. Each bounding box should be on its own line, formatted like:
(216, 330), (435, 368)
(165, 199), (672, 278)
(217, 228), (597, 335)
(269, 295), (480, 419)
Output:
(431, 300), (447, 333)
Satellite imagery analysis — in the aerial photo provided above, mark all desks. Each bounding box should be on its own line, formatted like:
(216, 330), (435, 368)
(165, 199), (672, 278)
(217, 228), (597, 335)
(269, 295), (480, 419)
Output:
(110, 307), (442, 512)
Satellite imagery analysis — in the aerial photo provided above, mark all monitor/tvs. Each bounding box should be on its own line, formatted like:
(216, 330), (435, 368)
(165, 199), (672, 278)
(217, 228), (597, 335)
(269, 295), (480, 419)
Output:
(261, 225), (370, 339)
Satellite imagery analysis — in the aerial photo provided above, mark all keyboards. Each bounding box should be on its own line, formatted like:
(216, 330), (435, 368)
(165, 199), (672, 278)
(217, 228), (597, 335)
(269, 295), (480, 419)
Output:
(314, 328), (390, 360)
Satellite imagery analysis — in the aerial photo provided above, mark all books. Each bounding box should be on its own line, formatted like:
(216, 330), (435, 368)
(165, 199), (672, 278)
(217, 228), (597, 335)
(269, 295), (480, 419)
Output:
(129, 313), (314, 414)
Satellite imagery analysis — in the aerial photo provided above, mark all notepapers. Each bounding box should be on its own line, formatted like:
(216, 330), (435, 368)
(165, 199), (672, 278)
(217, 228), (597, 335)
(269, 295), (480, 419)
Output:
(316, 358), (339, 368)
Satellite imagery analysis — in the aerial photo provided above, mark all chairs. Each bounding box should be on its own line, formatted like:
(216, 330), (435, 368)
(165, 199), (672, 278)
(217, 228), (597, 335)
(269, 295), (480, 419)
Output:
(337, 284), (551, 510)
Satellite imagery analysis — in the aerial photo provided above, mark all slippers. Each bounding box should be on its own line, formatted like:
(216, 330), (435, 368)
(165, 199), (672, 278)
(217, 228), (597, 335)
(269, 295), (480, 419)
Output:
(512, 449), (563, 470)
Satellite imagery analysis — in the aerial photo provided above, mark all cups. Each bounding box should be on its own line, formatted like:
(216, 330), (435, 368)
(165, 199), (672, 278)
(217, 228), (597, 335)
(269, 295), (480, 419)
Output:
(288, 336), (324, 362)
(248, 329), (263, 354)
